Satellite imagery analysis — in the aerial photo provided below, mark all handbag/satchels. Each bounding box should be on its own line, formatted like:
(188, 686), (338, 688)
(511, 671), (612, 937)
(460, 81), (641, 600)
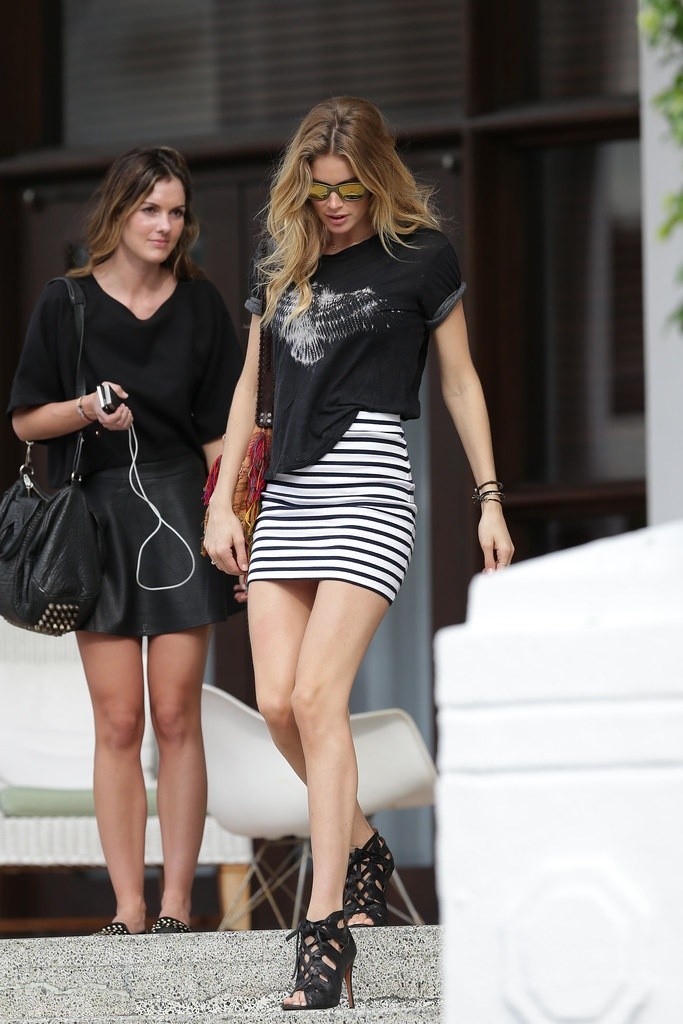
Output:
(0, 473), (102, 637)
(198, 416), (273, 583)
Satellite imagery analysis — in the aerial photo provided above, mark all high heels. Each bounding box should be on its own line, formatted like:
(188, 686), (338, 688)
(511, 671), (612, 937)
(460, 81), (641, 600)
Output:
(342, 828), (394, 927)
(281, 909), (357, 1010)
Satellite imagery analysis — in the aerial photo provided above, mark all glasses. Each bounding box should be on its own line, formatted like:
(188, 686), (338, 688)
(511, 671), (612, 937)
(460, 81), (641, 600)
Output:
(306, 181), (370, 201)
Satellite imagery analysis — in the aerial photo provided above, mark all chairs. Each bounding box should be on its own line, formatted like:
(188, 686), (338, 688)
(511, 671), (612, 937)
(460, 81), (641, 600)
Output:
(199, 682), (439, 933)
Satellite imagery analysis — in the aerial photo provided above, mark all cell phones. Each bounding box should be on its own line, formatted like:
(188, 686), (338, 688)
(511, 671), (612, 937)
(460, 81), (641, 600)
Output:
(96, 384), (120, 414)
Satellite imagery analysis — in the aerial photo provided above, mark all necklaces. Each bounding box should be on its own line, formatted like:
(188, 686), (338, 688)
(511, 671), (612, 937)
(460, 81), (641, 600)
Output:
(331, 236), (363, 250)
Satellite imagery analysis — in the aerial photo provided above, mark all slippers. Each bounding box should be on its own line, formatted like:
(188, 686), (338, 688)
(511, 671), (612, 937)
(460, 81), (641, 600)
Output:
(151, 916), (191, 933)
(92, 922), (146, 936)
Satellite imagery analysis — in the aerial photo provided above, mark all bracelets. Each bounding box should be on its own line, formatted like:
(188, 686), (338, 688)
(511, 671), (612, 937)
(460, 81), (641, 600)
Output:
(472, 481), (506, 504)
(77, 394), (93, 422)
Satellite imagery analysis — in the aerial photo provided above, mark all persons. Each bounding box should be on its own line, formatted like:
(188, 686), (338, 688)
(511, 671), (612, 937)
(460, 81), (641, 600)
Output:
(6, 141), (245, 933)
(200, 97), (516, 1010)
